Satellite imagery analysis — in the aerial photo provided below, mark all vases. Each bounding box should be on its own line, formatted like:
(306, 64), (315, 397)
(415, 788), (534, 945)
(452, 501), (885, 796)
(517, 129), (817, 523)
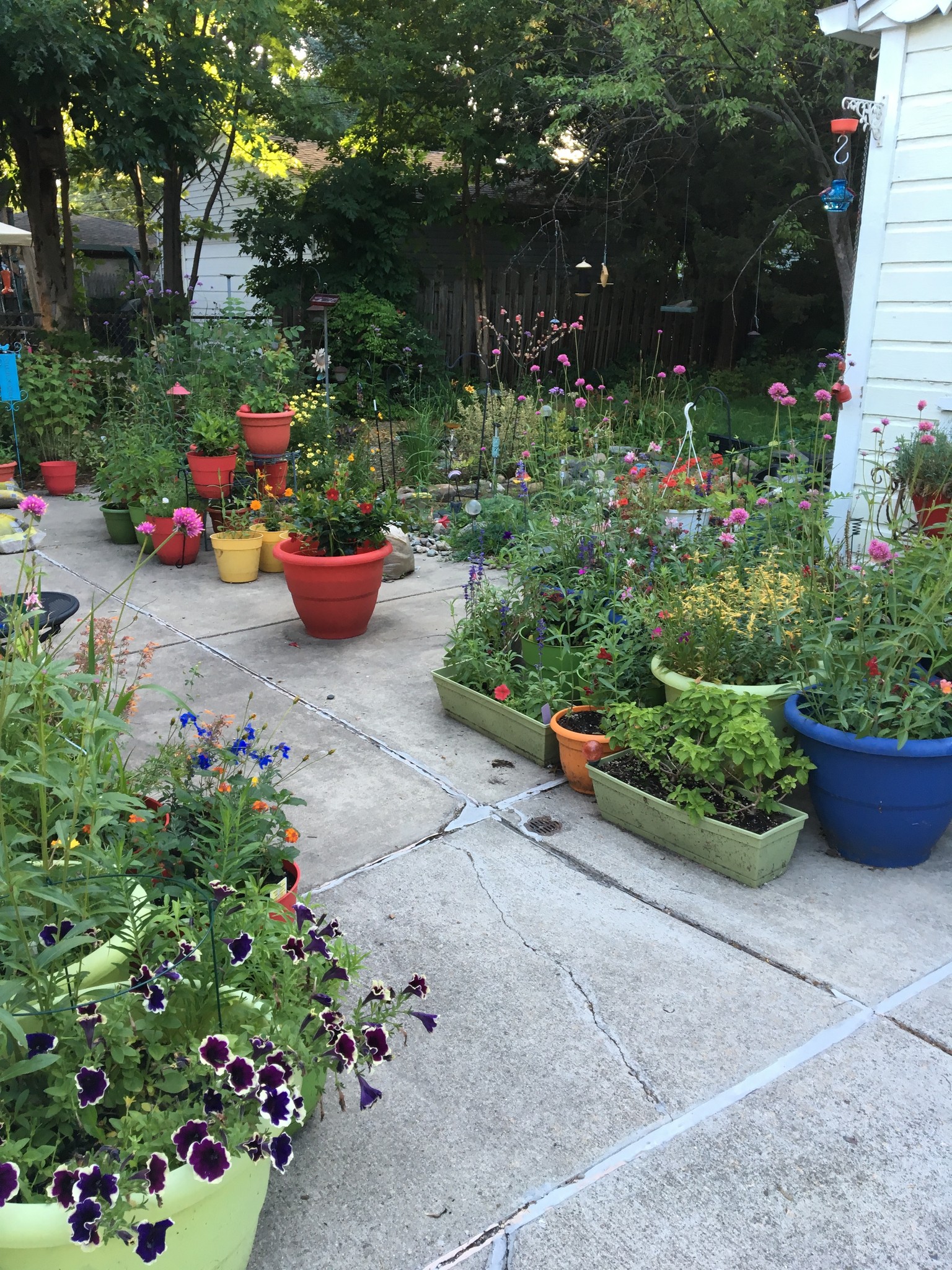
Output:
(785, 683), (950, 866)
(100, 503), (136, 546)
(254, 849), (300, 926)
(126, 501), (153, 552)
(210, 528), (264, 584)
(39, 461), (78, 493)
(0, 823), (450, 1270)
(23, 854), (151, 990)
(246, 521), (300, 573)
(2, 459), (19, 481)
(146, 511), (204, 564)
(580, 744), (808, 884)
(273, 530), (394, 644)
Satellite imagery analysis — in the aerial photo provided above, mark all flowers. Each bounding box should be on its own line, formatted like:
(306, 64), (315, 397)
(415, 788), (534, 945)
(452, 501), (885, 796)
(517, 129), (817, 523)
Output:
(432, 343), (951, 831)
(0, 231), (391, 549)
(0, 655), (201, 989)
(1, 481), (203, 771)
(142, 658), (309, 896)
